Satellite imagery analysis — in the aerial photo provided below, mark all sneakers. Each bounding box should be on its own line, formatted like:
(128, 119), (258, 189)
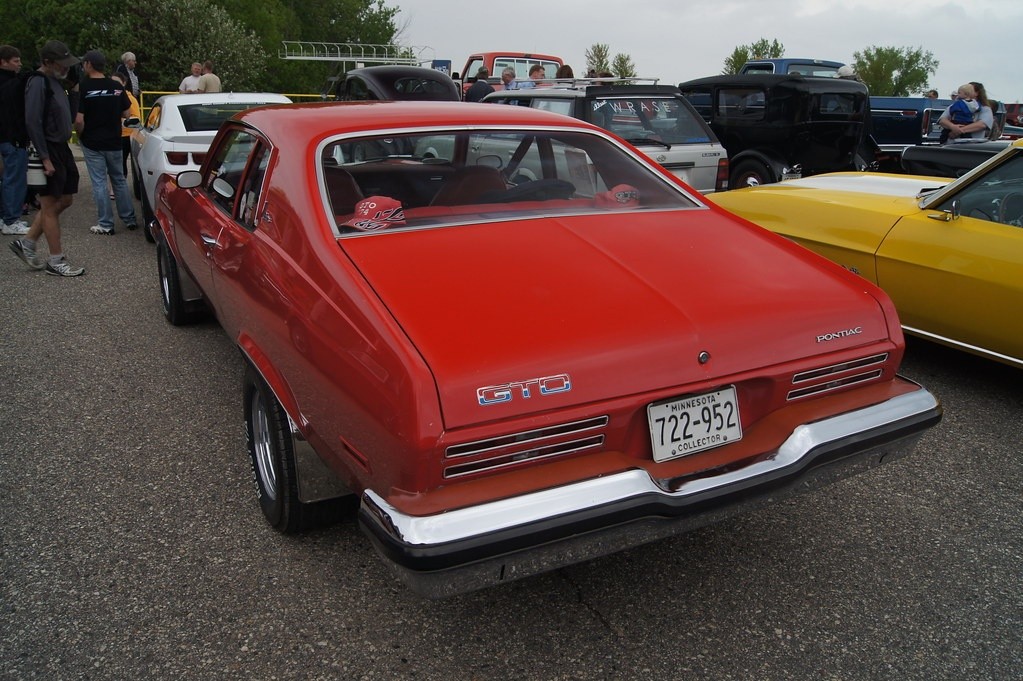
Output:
(126, 222), (138, 230)
(90, 224), (116, 236)
(43, 255), (85, 277)
(1, 218), (30, 234)
(9, 240), (46, 269)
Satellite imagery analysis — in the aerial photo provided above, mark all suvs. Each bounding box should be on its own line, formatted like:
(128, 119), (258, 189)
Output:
(413, 76), (728, 198)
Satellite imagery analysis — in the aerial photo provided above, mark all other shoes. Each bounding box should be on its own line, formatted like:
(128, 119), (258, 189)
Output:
(109, 188), (115, 200)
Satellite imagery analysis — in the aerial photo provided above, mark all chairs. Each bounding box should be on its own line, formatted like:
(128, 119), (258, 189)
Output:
(428, 164), (508, 207)
(324, 165), (366, 216)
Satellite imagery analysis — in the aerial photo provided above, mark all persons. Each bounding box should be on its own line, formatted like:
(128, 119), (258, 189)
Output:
(179, 62), (202, 94)
(117, 52), (140, 97)
(936, 80), (994, 145)
(498, 65), (521, 106)
(465, 65), (498, 103)
(110, 72), (141, 200)
(73, 51), (139, 235)
(0, 45), (41, 236)
(519, 63), (545, 87)
(922, 90), (938, 135)
(8, 39), (85, 277)
(596, 72), (614, 85)
(556, 65), (574, 87)
(195, 59), (222, 93)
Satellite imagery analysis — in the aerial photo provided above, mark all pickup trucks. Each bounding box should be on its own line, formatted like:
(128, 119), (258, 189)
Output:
(686, 58), (1007, 151)
(451, 52), (677, 132)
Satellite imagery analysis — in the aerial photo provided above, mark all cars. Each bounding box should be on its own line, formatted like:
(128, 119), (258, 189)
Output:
(334, 65), (461, 163)
(648, 74), (883, 190)
(124, 93), (345, 244)
(705, 137), (1023, 369)
(900, 139), (1023, 181)
(151, 99), (943, 598)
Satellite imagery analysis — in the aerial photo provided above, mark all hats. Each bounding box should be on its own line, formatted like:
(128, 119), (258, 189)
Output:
(594, 183), (642, 211)
(42, 40), (81, 66)
(339, 195), (408, 231)
